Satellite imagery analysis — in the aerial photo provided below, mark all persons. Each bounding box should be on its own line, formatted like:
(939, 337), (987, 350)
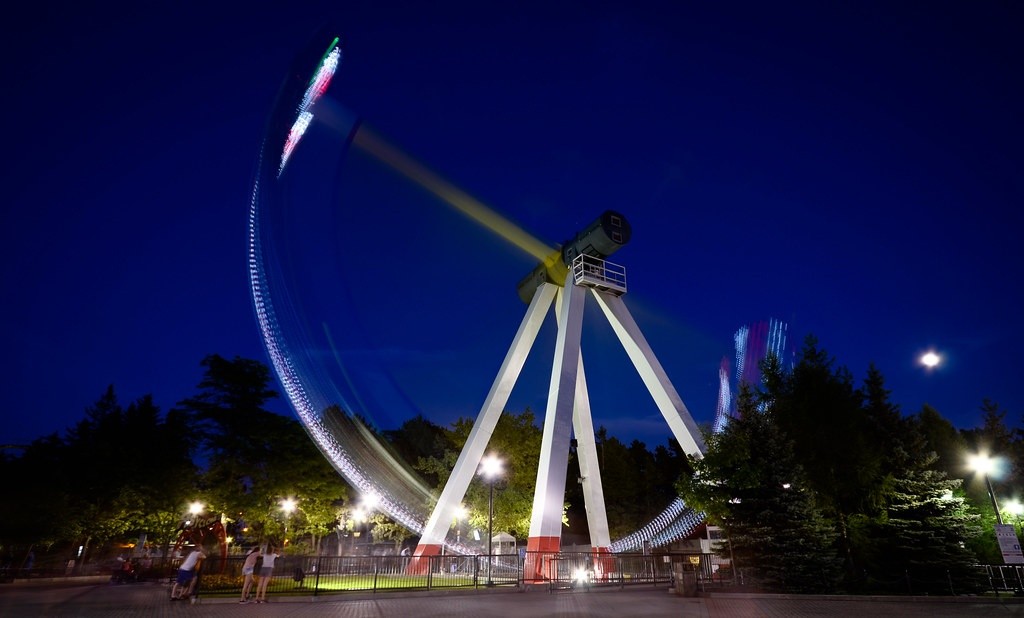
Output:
(117, 544), (280, 603)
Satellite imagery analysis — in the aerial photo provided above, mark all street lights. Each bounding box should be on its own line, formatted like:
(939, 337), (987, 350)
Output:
(476, 452), (505, 586)
(962, 450), (1024, 596)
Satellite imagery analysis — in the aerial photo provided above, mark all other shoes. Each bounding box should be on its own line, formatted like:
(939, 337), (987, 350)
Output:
(179, 594), (189, 600)
(255, 600), (260, 603)
(170, 596), (180, 601)
(261, 600), (268, 604)
(239, 600), (248, 604)
